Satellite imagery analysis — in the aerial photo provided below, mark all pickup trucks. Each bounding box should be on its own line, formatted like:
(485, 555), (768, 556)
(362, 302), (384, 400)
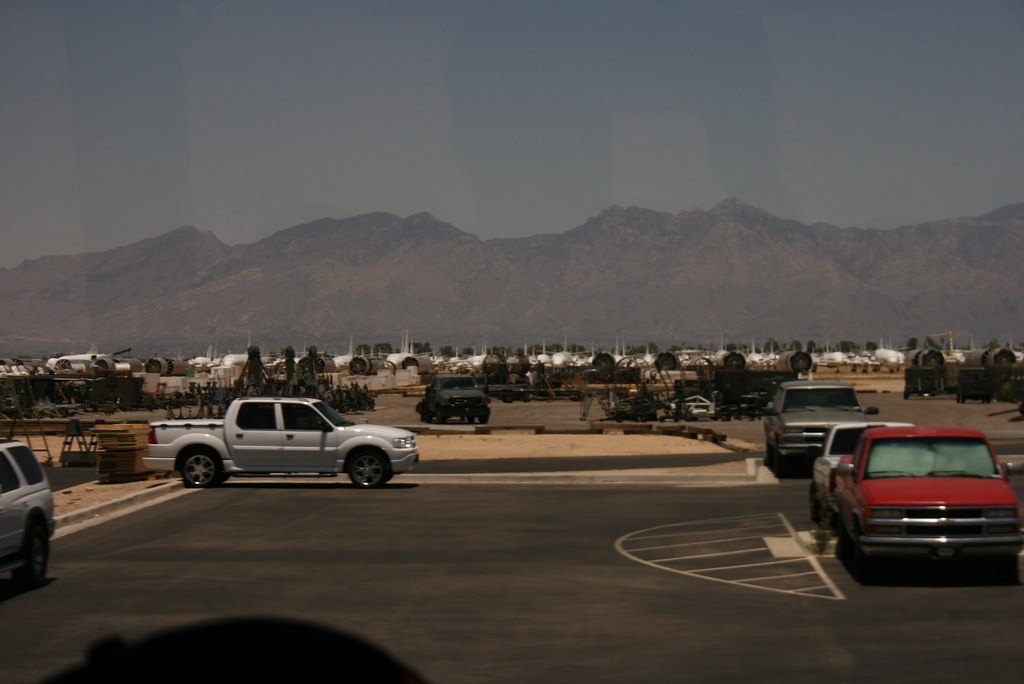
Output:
(141, 398), (419, 490)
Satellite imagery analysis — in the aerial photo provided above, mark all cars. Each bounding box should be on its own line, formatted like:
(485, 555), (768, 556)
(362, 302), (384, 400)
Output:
(810, 421), (914, 530)
(0, 440), (58, 588)
(835, 424), (1024, 587)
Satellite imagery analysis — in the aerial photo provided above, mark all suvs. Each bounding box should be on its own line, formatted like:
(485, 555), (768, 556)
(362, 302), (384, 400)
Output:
(425, 373), (491, 424)
(761, 379), (881, 472)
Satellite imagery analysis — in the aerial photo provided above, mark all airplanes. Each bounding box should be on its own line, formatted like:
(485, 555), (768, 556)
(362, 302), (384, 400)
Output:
(0, 330), (1024, 377)
